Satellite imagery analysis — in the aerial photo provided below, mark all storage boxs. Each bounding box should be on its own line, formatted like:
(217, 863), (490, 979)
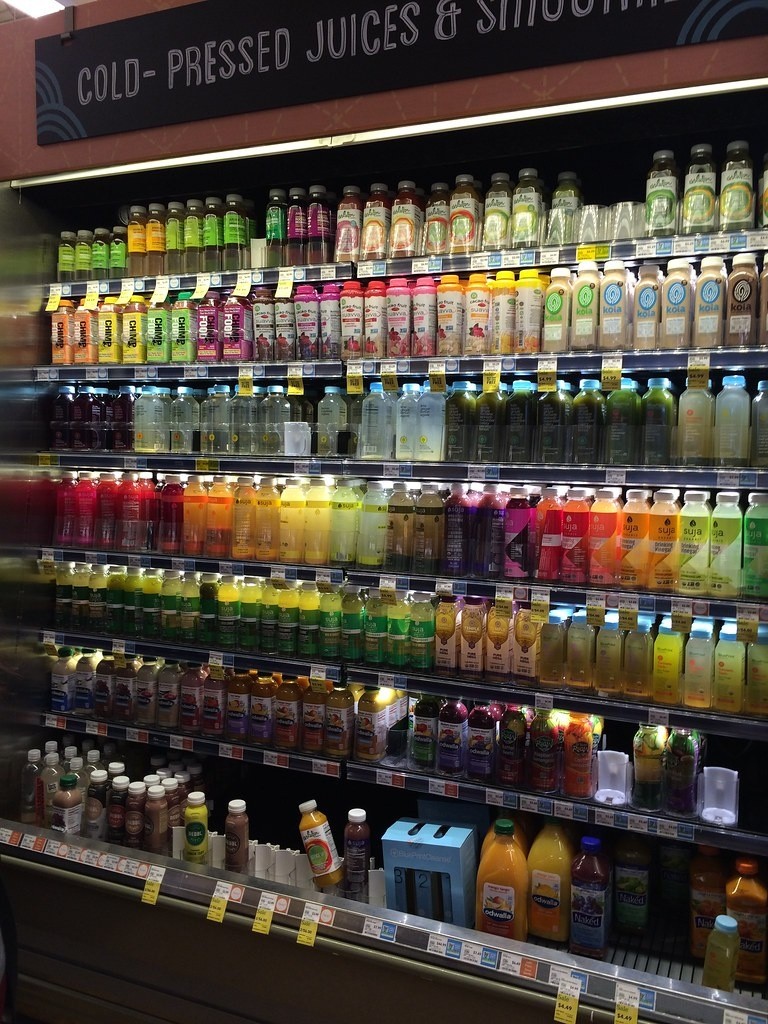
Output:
(380, 797), (493, 929)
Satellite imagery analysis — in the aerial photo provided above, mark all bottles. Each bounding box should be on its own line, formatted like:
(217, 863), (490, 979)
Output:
(24, 139), (768, 994)
